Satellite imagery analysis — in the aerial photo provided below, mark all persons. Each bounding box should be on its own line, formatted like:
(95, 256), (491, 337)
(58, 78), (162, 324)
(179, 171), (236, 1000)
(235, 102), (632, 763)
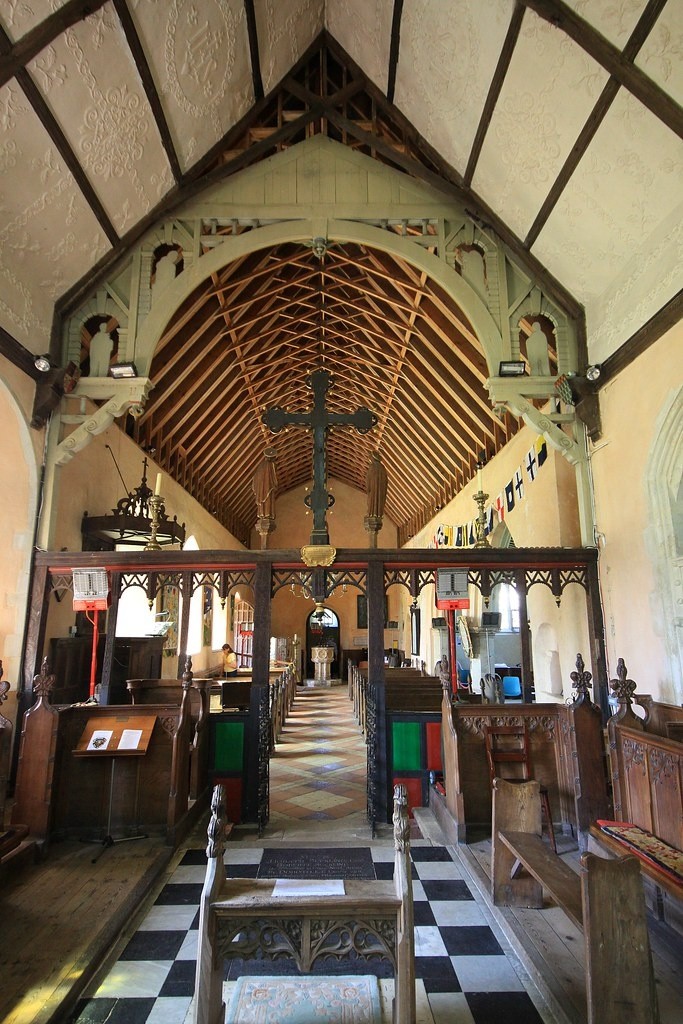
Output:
(221, 644), (238, 678)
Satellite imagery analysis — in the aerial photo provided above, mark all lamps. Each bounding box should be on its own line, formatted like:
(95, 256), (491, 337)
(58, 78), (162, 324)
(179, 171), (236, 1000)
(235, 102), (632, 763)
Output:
(585, 362), (600, 381)
(108, 360), (137, 379)
(498, 361), (526, 378)
(35, 352), (53, 372)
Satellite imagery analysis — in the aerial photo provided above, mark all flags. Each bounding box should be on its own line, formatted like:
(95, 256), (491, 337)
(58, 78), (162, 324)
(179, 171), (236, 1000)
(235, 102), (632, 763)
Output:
(433, 434), (547, 549)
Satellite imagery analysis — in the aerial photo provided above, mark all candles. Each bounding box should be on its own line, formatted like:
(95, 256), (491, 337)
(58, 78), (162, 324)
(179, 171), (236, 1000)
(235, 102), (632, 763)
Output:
(478, 469), (482, 491)
(154, 473), (163, 497)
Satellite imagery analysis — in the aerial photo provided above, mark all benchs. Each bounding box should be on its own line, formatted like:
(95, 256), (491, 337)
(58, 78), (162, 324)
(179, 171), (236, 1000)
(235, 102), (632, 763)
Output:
(198, 664), (296, 745)
(588, 820), (683, 911)
(348, 658), (445, 742)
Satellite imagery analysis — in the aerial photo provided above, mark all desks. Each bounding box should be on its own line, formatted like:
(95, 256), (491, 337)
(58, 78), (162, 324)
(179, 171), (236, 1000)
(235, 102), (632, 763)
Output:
(192, 783), (417, 1024)
(490, 778), (658, 1024)
